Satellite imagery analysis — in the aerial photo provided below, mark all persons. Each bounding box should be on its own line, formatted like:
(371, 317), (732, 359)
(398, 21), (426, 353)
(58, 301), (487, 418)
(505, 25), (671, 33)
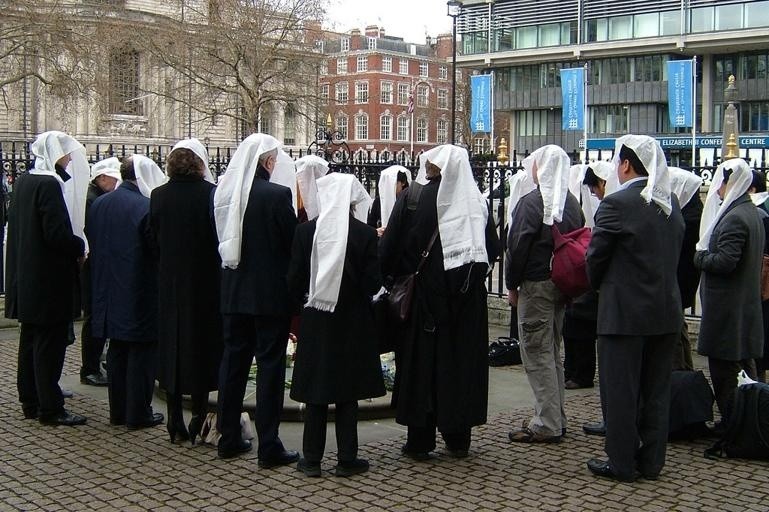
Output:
(583, 132), (685, 483)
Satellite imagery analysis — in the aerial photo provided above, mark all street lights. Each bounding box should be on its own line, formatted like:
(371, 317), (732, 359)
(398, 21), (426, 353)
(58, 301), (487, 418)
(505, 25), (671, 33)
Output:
(20, 22), (30, 156)
(184, 36), (194, 140)
(446, 0), (462, 146)
(311, 61), (320, 150)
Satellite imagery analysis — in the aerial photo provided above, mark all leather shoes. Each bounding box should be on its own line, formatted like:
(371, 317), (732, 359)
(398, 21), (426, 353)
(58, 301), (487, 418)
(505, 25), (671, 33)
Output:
(126, 411), (165, 431)
(216, 439), (253, 459)
(56, 386), (74, 400)
(586, 457), (662, 485)
(257, 450), (302, 470)
(582, 421), (608, 436)
(80, 368), (110, 387)
(44, 411), (88, 427)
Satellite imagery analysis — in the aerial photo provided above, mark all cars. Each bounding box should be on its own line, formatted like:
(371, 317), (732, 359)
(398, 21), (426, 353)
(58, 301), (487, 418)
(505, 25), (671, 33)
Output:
(482, 178), (509, 229)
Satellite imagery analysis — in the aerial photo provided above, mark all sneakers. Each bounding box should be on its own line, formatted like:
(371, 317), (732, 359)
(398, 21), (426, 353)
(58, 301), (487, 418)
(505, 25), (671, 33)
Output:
(446, 442), (471, 460)
(508, 416), (568, 445)
(296, 453), (324, 480)
(334, 459), (371, 478)
(400, 441), (431, 461)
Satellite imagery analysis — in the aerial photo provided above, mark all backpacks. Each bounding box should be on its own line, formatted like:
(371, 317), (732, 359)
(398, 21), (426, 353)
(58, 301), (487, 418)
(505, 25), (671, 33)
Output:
(704, 379), (769, 463)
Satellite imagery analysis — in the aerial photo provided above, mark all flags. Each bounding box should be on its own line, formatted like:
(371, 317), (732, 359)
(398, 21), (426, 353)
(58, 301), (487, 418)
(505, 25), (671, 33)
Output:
(407, 85), (415, 114)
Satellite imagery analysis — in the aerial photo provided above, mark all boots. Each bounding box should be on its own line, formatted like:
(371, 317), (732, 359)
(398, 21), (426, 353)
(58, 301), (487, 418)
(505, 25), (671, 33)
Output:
(164, 380), (190, 445)
(187, 381), (212, 447)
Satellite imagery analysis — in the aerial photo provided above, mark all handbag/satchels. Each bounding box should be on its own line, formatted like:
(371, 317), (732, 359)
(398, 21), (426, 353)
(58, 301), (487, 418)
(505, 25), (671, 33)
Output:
(663, 364), (718, 445)
(760, 254), (769, 303)
(485, 336), (523, 368)
(386, 274), (419, 325)
(547, 222), (593, 300)
